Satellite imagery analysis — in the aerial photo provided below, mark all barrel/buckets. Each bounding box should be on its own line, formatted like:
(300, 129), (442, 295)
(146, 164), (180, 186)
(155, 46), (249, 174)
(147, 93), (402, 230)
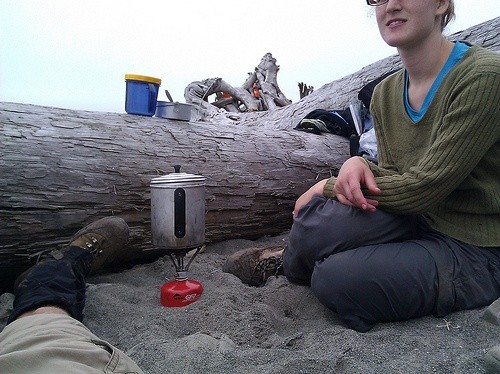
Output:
(125, 74), (161, 116)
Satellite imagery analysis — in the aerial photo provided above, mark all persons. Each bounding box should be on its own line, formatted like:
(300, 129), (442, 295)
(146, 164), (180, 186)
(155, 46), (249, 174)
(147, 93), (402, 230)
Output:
(221, 0), (500, 332)
(0, 216), (148, 374)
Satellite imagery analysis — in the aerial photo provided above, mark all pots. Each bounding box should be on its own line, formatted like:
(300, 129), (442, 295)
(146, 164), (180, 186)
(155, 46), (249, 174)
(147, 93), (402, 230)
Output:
(150, 165), (205, 249)
(155, 101), (194, 120)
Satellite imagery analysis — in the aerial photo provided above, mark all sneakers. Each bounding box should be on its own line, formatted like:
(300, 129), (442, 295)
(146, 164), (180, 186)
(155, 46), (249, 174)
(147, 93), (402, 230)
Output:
(37, 216), (130, 274)
(222, 245), (287, 287)
